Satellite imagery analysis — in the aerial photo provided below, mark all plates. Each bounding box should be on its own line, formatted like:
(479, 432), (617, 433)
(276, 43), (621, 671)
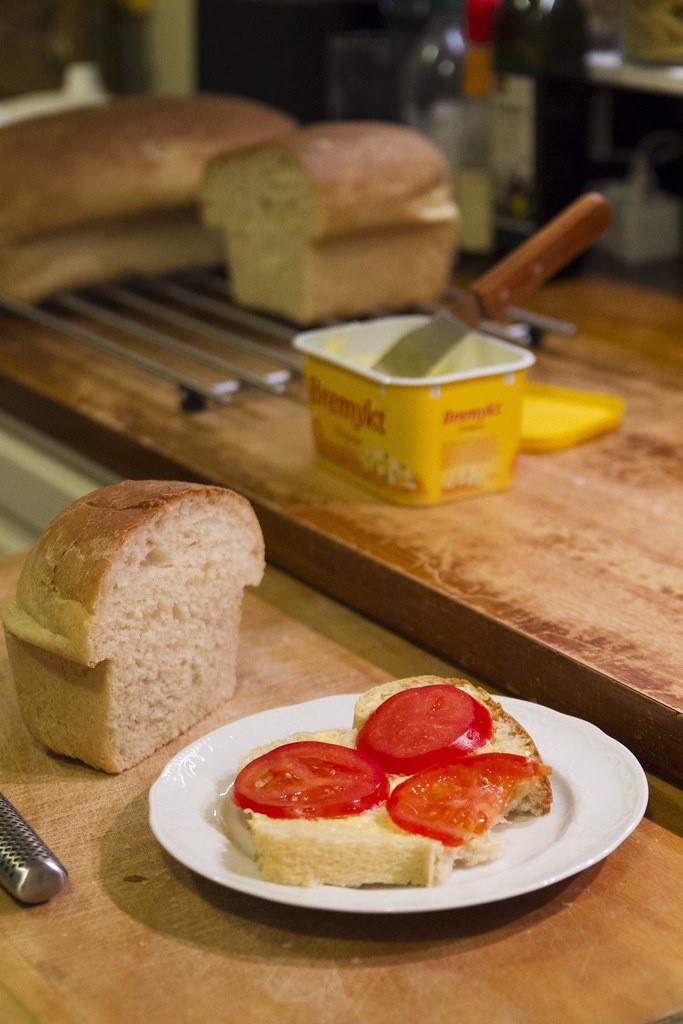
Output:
(146, 692), (650, 915)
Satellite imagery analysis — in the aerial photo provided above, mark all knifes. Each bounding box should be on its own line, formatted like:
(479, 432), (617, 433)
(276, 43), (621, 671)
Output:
(370, 194), (614, 378)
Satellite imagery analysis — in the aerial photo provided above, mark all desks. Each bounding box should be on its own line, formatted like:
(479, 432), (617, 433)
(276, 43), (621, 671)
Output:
(0, 260), (683, 777)
(586, 51), (683, 94)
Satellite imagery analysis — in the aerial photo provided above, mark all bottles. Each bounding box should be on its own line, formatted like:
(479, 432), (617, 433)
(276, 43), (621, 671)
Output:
(398, 0), (498, 274)
(489, 0), (589, 285)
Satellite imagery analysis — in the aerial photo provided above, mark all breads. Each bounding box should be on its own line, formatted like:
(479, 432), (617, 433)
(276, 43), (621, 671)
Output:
(237, 729), (443, 889)
(2, 481), (267, 773)
(0, 93), (294, 299)
(199, 121), (456, 320)
(354, 676), (553, 816)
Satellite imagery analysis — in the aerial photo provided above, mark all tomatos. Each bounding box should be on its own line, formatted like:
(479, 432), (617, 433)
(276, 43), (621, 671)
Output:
(358, 682), (492, 774)
(232, 741), (391, 817)
(390, 755), (551, 845)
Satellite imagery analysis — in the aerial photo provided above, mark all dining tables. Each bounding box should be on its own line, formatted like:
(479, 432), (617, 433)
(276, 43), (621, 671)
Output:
(0, 514), (682, 1023)
